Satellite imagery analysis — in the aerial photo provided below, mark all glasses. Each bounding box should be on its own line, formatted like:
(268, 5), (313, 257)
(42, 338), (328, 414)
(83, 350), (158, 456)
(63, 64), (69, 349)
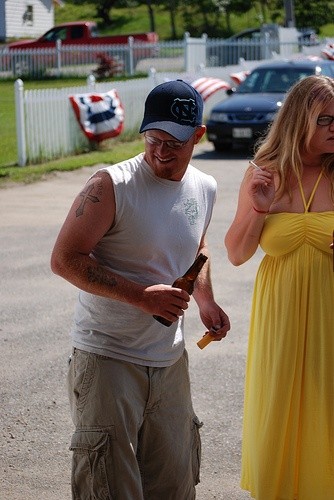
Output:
(317, 115), (334, 126)
(144, 132), (195, 149)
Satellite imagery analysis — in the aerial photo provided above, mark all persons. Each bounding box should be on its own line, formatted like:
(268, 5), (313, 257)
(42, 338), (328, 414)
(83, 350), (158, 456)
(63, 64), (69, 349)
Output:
(225, 76), (334, 500)
(92, 52), (122, 80)
(51, 79), (231, 500)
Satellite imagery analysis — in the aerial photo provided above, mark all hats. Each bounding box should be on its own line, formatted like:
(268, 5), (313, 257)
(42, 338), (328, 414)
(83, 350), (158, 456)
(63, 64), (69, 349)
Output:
(140, 78), (204, 144)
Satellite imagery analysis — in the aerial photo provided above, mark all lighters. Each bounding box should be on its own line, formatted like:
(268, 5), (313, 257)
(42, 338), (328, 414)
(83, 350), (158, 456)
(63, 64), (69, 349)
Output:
(197, 333), (216, 349)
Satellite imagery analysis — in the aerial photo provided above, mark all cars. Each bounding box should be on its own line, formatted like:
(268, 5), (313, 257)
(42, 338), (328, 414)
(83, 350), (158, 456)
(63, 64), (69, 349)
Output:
(206, 29), (319, 67)
(207, 60), (334, 152)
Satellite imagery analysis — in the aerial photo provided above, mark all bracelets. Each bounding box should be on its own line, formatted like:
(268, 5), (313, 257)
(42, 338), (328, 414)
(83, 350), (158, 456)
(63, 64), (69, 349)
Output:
(254, 206), (269, 214)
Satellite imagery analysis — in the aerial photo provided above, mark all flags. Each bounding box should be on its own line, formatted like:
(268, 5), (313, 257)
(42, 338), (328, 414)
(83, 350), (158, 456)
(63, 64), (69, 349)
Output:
(190, 71), (250, 101)
(69, 88), (123, 140)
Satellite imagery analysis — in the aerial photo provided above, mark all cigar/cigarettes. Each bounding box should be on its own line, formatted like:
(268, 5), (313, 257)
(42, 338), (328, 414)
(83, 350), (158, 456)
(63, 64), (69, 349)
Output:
(249, 161), (263, 172)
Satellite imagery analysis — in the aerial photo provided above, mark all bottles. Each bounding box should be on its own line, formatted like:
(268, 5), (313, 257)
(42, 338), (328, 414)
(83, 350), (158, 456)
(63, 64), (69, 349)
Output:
(153, 253), (208, 327)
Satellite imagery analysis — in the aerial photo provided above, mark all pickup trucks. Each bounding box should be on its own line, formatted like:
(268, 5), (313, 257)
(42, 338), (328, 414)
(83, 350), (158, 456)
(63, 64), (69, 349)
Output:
(0, 22), (158, 78)
